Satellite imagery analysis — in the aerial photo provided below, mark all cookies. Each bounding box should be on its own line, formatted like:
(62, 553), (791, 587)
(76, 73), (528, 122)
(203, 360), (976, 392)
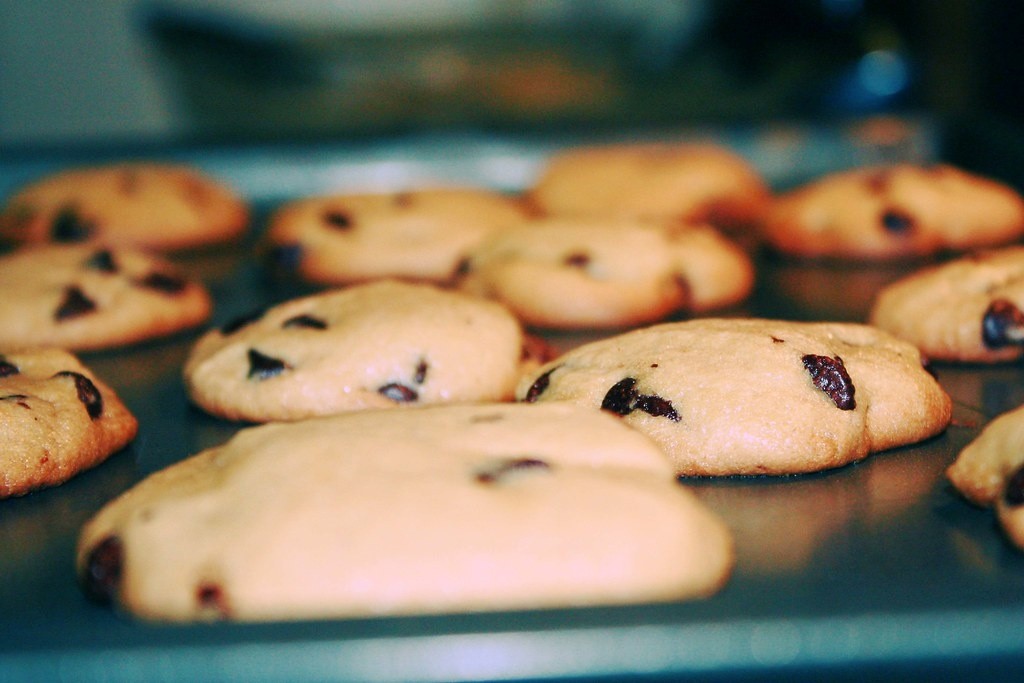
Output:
(0, 139), (1024, 623)
